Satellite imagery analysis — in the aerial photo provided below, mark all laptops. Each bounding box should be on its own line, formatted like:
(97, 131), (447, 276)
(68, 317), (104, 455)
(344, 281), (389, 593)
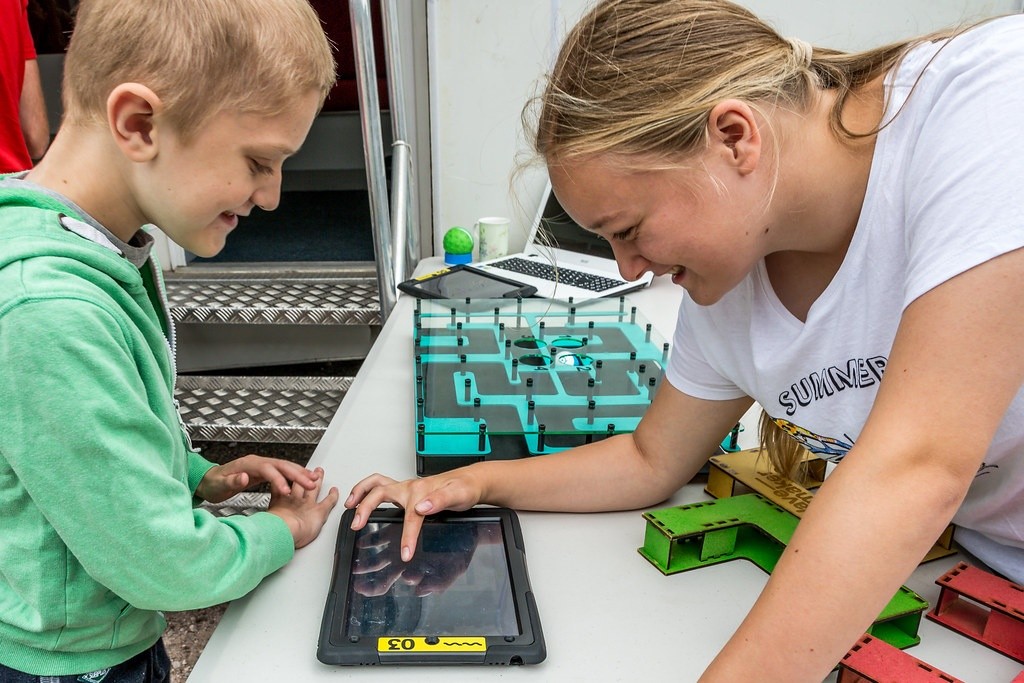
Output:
(464, 173), (655, 310)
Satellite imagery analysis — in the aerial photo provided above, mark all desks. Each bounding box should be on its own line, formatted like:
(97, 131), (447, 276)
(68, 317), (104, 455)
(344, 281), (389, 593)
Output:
(187, 255), (1024, 682)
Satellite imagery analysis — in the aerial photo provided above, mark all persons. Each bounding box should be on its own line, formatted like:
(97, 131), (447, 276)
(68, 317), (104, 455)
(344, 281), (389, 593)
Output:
(0, 2), (342, 682)
(341, 1), (1024, 683)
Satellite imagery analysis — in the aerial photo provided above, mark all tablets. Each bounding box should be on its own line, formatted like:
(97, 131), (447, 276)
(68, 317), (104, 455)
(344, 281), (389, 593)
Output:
(397, 263), (538, 314)
(316, 507), (547, 668)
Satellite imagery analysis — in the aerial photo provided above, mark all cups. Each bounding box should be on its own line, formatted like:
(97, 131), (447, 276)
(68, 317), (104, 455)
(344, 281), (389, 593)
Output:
(473, 217), (510, 262)
